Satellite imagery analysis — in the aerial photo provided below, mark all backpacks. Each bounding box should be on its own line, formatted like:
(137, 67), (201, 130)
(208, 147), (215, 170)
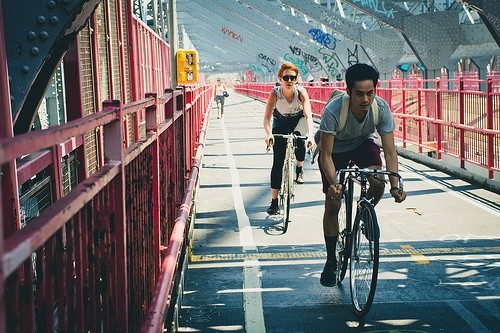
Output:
(311, 89), (378, 165)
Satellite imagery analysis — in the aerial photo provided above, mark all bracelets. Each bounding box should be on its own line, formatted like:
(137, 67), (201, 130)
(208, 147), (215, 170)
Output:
(390, 187), (399, 194)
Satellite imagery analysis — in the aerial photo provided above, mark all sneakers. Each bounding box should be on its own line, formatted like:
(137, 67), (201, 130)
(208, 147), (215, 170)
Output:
(320, 259), (337, 287)
(267, 198), (279, 214)
(361, 208), (380, 241)
(296, 165), (304, 184)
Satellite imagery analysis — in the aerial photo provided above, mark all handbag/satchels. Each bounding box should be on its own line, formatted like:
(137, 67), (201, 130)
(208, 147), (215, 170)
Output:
(223, 93), (229, 97)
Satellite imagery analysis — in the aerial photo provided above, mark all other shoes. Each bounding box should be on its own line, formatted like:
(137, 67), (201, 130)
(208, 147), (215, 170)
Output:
(217, 116), (220, 119)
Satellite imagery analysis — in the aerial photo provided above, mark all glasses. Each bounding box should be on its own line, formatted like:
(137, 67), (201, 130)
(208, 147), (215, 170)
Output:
(217, 80), (220, 81)
(280, 75), (296, 82)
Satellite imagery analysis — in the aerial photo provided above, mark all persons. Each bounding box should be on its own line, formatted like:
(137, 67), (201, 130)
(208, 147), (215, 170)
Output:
(316, 64), (407, 287)
(213, 78), (227, 119)
(263, 64), (316, 215)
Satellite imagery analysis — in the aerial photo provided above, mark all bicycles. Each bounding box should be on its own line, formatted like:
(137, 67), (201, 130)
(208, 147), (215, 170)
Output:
(265, 131), (309, 233)
(331, 159), (403, 318)
